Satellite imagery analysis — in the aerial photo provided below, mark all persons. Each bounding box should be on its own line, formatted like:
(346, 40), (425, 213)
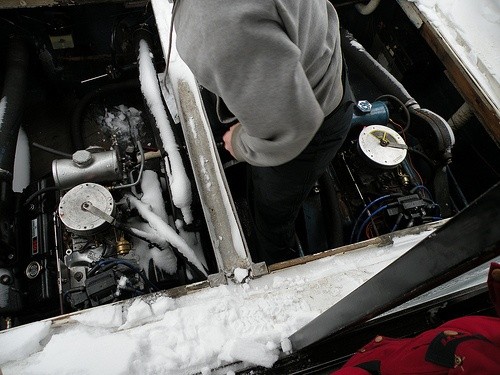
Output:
(327, 258), (499, 375)
(172, 0), (357, 268)
